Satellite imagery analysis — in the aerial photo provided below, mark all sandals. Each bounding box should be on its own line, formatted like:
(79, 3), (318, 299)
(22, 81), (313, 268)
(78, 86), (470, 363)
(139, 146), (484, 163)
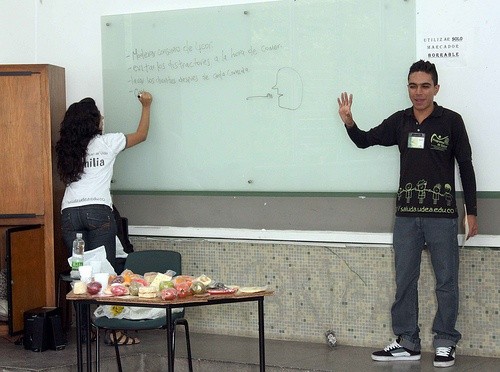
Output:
(108, 331), (138, 345)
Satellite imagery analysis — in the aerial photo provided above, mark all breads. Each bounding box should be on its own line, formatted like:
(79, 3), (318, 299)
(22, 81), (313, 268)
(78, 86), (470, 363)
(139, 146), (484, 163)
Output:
(73, 282), (88, 294)
(138, 286), (156, 298)
(150, 271), (173, 291)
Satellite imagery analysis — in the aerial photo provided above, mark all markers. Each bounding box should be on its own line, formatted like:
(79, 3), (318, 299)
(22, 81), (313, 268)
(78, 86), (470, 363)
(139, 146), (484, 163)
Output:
(138, 94), (142, 98)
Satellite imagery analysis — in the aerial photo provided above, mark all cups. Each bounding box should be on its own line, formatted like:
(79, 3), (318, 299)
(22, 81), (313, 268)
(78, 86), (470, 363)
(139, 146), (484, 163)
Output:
(90, 261), (101, 275)
(70, 281), (87, 295)
(79, 265), (92, 282)
(94, 273), (108, 294)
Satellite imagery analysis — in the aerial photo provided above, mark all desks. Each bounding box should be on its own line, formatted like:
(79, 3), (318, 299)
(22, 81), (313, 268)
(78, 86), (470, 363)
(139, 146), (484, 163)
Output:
(65, 290), (274, 372)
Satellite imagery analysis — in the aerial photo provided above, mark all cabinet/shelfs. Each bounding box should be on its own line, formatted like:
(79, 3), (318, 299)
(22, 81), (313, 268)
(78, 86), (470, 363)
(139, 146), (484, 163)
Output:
(0, 65), (72, 340)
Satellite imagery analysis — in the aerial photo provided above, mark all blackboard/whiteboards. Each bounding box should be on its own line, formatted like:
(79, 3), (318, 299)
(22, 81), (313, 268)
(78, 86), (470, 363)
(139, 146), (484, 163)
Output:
(99, 0), (417, 198)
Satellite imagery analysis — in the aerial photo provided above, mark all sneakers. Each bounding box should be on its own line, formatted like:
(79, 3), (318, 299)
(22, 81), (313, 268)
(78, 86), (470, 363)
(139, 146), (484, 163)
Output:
(371, 341), (421, 361)
(433, 345), (455, 368)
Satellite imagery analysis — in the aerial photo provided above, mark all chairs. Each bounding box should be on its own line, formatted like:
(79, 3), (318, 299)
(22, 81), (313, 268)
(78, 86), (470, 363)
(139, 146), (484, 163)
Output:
(90, 250), (193, 372)
(57, 275), (80, 307)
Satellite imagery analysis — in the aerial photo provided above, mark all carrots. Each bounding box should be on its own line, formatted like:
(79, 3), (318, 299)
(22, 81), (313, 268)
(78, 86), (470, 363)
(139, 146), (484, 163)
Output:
(124, 271), (133, 283)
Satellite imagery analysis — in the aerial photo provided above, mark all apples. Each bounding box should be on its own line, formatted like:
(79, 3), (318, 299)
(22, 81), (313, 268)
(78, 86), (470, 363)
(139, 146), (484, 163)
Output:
(129, 282), (142, 295)
(158, 281), (206, 300)
(87, 282), (102, 295)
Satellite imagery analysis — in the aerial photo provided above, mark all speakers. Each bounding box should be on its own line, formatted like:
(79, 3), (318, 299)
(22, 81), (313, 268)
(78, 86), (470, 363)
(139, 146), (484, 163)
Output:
(23, 306), (59, 352)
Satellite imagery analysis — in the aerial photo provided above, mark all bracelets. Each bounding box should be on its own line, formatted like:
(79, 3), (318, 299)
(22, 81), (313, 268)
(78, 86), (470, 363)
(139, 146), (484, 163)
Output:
(346, 122), (354, 127)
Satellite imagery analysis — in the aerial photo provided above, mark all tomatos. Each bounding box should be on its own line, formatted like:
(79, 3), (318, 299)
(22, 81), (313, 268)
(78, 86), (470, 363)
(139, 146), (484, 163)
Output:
(175, 284), (189, 298)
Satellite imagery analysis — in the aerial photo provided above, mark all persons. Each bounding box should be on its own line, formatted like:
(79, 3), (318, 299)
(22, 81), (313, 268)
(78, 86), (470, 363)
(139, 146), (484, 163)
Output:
(55, 90), (153, 345)
(337, 60), (479, 367)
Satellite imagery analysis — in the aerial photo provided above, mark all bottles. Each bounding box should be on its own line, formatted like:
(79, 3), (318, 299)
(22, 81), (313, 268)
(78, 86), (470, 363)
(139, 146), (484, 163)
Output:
(71, 232), (85, 279)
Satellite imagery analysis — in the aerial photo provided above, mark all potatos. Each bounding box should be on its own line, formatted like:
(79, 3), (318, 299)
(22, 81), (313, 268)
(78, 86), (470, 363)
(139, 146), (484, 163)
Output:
(110, 285), (127, 295)
